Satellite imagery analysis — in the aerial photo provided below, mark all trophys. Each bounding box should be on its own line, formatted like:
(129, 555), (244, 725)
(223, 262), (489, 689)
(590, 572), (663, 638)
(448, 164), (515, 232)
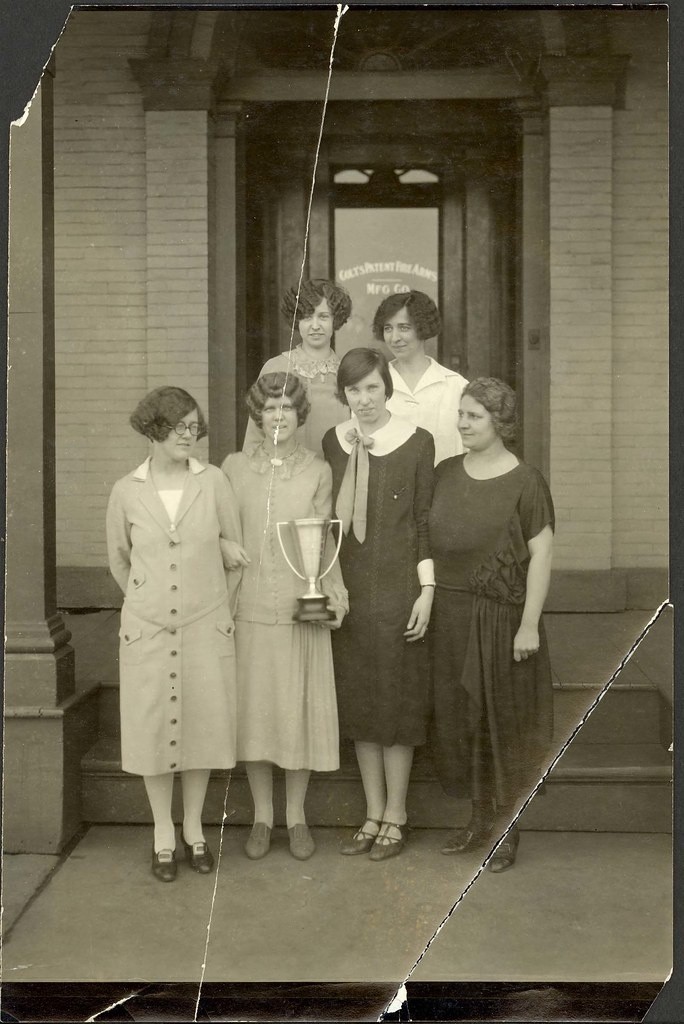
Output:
(275, 519), (344, 624)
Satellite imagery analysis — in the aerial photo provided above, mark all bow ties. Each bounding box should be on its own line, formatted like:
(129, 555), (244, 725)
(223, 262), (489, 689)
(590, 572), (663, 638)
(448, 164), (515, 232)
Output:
(335, 428), (374, 544)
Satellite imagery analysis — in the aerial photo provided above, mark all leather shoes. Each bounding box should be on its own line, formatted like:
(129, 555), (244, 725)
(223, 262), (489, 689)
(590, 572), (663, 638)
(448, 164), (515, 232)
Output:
(440, 823), (496, 854)
(180, 830), (214, 874)
(152, 841), (177, 881)
(488, 825), (520, 873)
(370, 817), (409, 860)
(341, 819), (381, 856)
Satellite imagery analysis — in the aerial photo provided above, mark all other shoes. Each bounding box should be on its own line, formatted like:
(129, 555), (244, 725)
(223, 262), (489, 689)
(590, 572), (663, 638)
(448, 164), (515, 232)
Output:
(246, 822), (271, 860)
(287, 823), (315, 860)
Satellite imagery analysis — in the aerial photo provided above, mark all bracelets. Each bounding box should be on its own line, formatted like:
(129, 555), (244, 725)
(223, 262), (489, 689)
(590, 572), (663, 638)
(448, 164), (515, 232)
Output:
(421, 582), (437, 587)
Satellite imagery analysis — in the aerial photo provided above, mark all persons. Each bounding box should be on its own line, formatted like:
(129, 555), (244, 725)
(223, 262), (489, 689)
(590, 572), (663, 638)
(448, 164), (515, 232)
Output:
(430, 376), (557, 871)
(322, 347), (436, 860)
(220, 372), (350, 860)
(243, 279), (354, 455)
(371, 291), (467, 468)
(106, 386), (242, 882)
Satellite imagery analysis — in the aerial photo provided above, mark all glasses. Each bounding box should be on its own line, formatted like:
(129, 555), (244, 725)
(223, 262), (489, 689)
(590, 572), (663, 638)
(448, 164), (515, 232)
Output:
(155, 421), (203, 436)
